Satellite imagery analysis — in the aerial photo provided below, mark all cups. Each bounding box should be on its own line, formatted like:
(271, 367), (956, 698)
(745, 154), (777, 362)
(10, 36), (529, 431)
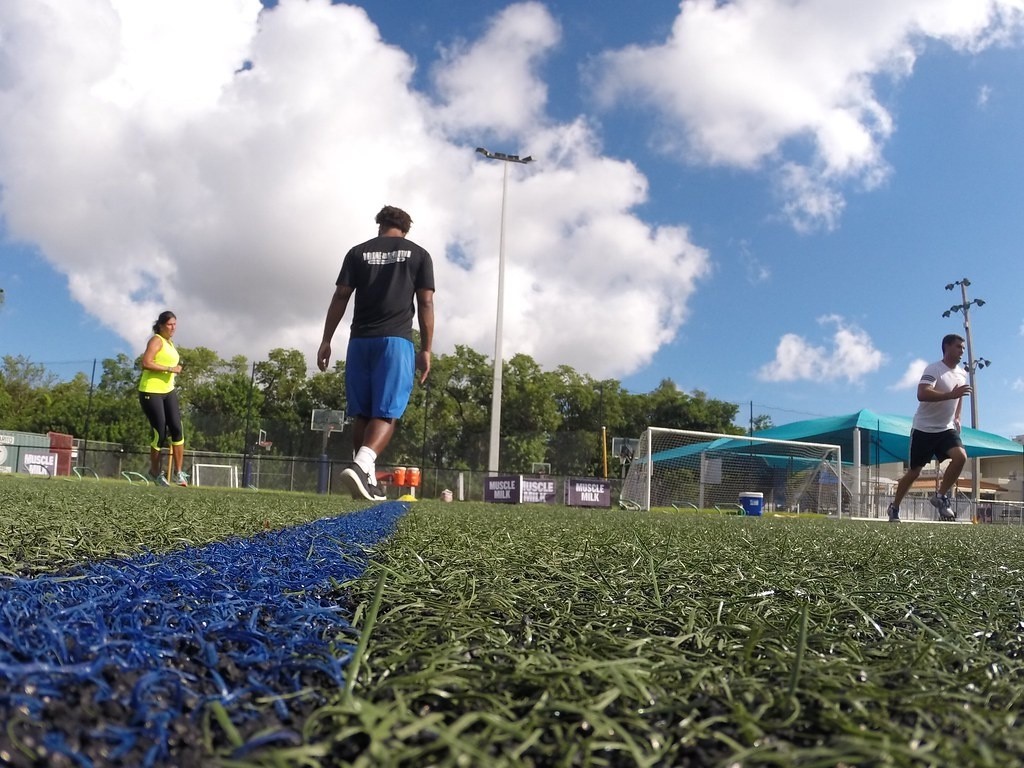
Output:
(440, 489), (454, 502)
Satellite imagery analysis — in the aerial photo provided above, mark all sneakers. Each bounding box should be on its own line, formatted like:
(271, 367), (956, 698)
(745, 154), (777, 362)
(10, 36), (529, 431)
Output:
(148, 468), (170, 487)
(175, 471), (188, 487)
(930, 492), (955, 517)
(887, 502), (900, 522)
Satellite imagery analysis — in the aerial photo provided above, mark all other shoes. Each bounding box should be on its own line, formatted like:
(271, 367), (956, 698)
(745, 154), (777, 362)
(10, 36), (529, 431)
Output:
(339, 462), (387, 501)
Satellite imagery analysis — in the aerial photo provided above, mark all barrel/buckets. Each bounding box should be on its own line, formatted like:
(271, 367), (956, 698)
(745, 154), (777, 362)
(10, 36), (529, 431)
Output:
(737, 491), (763, 516)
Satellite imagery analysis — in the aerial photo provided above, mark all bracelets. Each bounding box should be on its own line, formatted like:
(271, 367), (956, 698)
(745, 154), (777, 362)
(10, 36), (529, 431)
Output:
(168, 366), (173, 373)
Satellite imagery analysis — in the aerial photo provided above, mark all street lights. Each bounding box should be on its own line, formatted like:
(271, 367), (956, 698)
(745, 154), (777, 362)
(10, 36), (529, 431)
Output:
(474, 146), (537, 480)
(941, 276), (992, 508)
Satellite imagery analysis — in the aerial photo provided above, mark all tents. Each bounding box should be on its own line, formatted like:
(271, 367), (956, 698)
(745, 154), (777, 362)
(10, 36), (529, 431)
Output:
(625, 408), (1024, 470)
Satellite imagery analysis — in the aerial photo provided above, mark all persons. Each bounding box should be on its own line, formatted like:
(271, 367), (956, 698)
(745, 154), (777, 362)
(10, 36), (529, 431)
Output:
(139, 311), (190, 487)
(317, 205), (435, 500)
(887, 334), (973, 523)
